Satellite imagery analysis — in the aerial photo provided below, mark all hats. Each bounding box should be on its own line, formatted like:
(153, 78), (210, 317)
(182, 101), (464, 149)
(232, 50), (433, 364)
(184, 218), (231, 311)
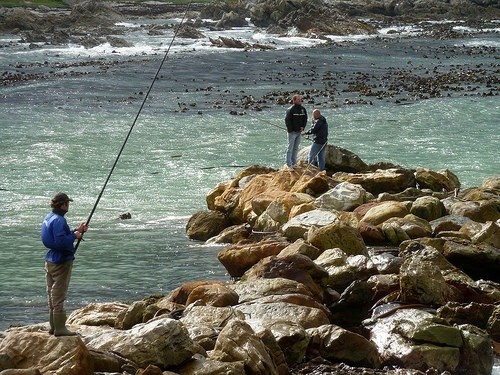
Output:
(50, 192), (74, 207)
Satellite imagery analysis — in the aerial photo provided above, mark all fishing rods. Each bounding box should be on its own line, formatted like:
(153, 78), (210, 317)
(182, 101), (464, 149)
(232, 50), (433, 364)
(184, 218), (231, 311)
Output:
(246, 114), (313, 141)
(296, 139), (331, 185)
(73, 0), (192, 255)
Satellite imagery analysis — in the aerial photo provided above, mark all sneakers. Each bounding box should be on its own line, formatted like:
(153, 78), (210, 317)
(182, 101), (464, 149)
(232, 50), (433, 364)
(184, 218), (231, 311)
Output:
(322, 170), (326, 174)
(287, 166), (295, 172)
(291, 164), (303, 169)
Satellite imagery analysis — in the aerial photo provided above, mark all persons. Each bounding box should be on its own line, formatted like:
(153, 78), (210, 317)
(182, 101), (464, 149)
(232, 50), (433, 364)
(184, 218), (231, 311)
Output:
(284, 95), (308, 171)
(301, 108), (329, 173)
(40, 192), (87, 336)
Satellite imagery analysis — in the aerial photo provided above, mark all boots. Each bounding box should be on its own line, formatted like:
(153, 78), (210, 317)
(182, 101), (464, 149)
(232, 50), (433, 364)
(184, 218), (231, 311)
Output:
(49, 311), (77, 337)
